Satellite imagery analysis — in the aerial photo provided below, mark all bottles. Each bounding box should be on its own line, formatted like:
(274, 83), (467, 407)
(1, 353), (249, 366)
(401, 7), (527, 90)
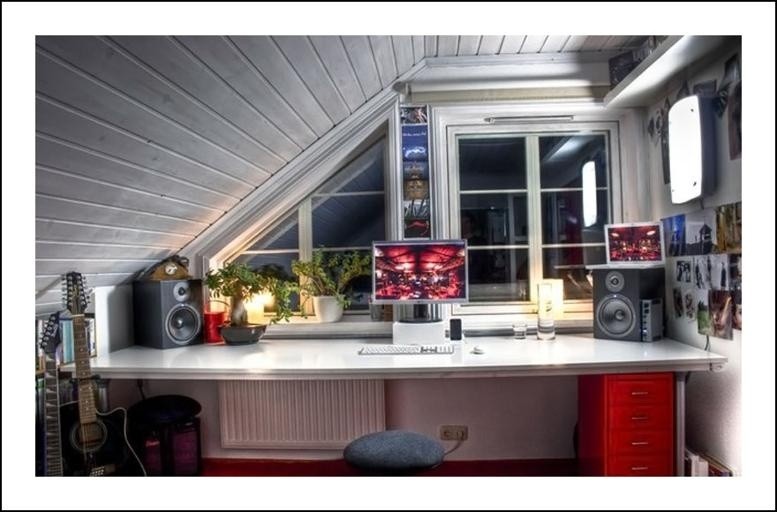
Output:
(537, 283), (555, 339)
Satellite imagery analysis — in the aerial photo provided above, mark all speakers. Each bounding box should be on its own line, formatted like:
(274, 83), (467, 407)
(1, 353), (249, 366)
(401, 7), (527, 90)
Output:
(591, 268), (666, 342)
(131, 278), (204, 349)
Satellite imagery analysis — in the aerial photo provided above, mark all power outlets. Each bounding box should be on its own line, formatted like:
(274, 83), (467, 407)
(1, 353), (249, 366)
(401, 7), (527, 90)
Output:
(440, 425), (469, 440)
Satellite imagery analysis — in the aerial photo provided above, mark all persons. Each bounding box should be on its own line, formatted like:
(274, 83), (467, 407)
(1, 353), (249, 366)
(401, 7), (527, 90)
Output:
(731, 290), (742, 331)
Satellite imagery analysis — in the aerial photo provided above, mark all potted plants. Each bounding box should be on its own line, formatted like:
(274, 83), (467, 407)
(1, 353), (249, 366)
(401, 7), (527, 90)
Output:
(205, 263), (292, 344)
(287, 244), (373, 322)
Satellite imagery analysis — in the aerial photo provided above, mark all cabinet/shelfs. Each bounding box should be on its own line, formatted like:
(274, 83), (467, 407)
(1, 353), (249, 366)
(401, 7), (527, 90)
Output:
(579, 372), (679, 476)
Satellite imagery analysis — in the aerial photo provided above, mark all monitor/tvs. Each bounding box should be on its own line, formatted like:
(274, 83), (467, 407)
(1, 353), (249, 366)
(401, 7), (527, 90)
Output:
(371, 239), (471, 323)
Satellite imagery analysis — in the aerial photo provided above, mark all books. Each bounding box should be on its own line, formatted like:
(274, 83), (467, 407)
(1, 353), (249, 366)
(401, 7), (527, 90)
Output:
(35, 311), (96, 373)
(684, 446), (733, 477)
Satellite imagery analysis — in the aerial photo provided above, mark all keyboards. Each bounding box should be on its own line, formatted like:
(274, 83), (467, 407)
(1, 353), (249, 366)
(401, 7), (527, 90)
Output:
(360, 345), (454, 355)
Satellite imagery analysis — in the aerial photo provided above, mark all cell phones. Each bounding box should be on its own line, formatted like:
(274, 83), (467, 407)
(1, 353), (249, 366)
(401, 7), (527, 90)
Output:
(450, 319), (462, 341)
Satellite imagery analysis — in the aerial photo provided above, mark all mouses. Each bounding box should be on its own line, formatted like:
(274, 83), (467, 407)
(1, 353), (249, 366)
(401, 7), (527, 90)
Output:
(474, 343), (485, 355)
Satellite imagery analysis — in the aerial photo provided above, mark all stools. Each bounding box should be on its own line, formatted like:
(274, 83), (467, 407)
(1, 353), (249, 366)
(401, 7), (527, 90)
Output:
(340, 430), (448, 476)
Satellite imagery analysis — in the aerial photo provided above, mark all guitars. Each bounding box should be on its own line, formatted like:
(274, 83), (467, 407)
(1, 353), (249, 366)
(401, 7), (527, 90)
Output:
(36, 310), (64, 478)
(60, 271), (148, 477)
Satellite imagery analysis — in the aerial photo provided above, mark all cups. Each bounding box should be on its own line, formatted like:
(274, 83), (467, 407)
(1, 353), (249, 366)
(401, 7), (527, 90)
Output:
(512, 322), (527, 340)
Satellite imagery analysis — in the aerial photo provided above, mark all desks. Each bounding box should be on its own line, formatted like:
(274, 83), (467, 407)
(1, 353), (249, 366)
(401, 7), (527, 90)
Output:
(53, 336), (727, 476)
